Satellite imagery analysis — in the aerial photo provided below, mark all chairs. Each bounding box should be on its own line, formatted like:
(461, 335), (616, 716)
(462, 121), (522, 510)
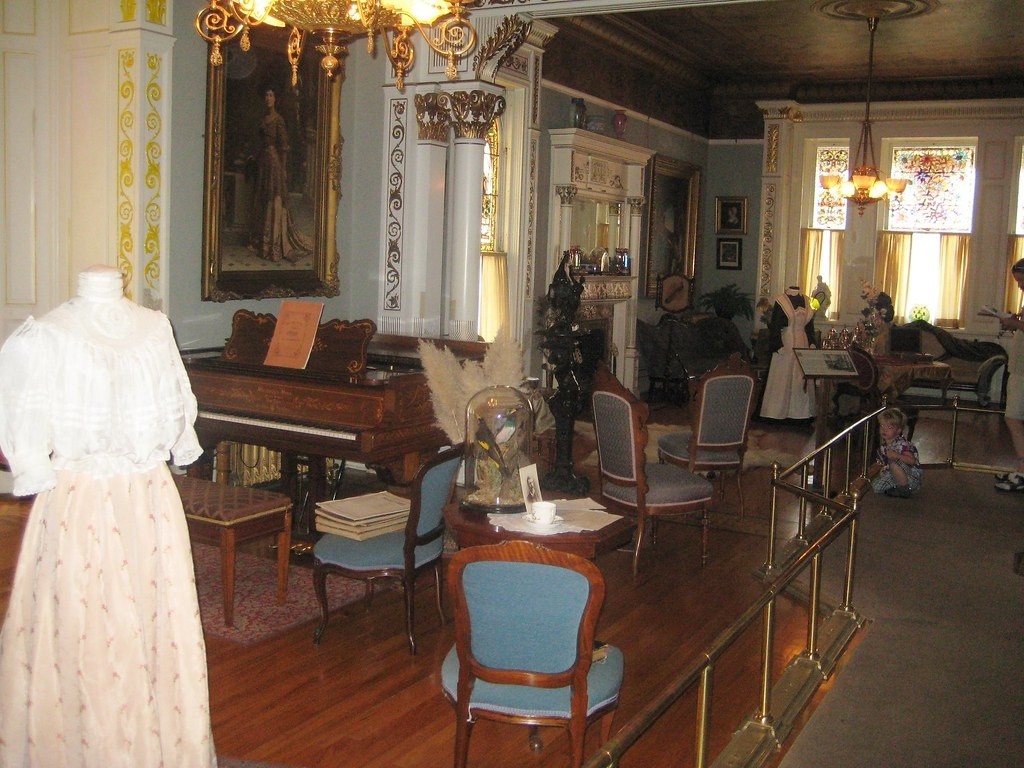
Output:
(440, 537), (625, 768)
(657, 353), (756, 529)
(592, 366), (714, 587)
(635, 310), (759, 406)
(312, 442), (467, 656)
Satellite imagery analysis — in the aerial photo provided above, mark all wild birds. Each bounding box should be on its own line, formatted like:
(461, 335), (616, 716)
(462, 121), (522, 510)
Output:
(494, 408), (521, 445)
(475, 413), (510, 474)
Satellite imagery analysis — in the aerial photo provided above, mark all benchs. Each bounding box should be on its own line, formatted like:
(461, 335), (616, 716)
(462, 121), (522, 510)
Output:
(886, 319), (1008, 405)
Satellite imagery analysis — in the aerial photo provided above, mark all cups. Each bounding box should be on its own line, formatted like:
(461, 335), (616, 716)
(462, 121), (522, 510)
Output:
(532, 502), (556, 524)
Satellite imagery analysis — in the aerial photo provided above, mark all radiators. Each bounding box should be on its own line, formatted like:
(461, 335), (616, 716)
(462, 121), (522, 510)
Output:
(230, 442), (335, 488)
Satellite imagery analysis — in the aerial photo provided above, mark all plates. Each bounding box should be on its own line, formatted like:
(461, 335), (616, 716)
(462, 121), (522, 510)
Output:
(522, 514), (564, 528)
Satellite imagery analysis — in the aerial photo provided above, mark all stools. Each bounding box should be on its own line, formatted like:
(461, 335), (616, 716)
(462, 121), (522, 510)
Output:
(174, 474), (295, 624)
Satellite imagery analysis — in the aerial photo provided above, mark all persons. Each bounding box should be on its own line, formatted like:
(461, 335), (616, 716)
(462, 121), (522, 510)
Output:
(994, 259), (1024, 490)
(872, 408), (920, 498)
(723, 245), (736, 262)
(244, 80), (315, 262)
(0, 271), (218, 768)
(611, 176), (623, 189)
(726, 206), (738, 224)
(751, 286), (817, 426)
(527, 477), (539, 502)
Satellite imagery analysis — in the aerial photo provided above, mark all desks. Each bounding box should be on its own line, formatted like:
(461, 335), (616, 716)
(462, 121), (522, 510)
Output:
(829, 361), (953, 419)
(440, 490), (638, 560)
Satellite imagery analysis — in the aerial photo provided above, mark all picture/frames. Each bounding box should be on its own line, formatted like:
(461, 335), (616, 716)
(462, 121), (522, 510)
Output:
(717, 238), (743, 270)
(640, 154), (702, 299)
(716, 196), (747, 236)
(200, 3), (348, 304)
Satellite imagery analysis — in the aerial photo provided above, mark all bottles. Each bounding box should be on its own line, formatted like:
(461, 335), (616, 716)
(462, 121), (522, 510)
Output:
(616, 248), (629, 271)
(561, 246), (582, 270)
(823, 322), (861, 350)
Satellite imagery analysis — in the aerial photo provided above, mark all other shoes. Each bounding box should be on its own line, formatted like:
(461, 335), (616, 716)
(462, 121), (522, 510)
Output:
(886, 484), (909, 498)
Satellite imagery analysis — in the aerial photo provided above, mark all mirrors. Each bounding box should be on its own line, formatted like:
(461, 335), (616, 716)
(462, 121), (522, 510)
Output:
(570, 195), (621, 269)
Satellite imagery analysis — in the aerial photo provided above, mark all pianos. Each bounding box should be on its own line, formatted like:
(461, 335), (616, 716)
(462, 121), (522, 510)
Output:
(176, 310), (490, 566)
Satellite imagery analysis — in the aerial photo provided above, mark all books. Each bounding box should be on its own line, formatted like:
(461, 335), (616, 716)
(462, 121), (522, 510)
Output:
(263, 301), (323, 370)
(315, 490), (412, 542)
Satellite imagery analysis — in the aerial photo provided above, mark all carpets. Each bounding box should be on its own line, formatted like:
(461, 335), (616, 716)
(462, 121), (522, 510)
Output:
(573, 415), (799, 469)
(778, 470), (1024, 768)
(191, 541), (382, 649)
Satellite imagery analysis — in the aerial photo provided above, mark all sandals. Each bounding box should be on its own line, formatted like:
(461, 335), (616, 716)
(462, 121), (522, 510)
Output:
(994, 476), (1024, 492)
(994, 472), (1016, 482)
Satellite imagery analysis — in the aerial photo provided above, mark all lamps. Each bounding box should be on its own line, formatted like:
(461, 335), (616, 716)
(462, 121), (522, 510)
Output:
(820, 2), (913, 217)
(196, 0), (477, 90)
